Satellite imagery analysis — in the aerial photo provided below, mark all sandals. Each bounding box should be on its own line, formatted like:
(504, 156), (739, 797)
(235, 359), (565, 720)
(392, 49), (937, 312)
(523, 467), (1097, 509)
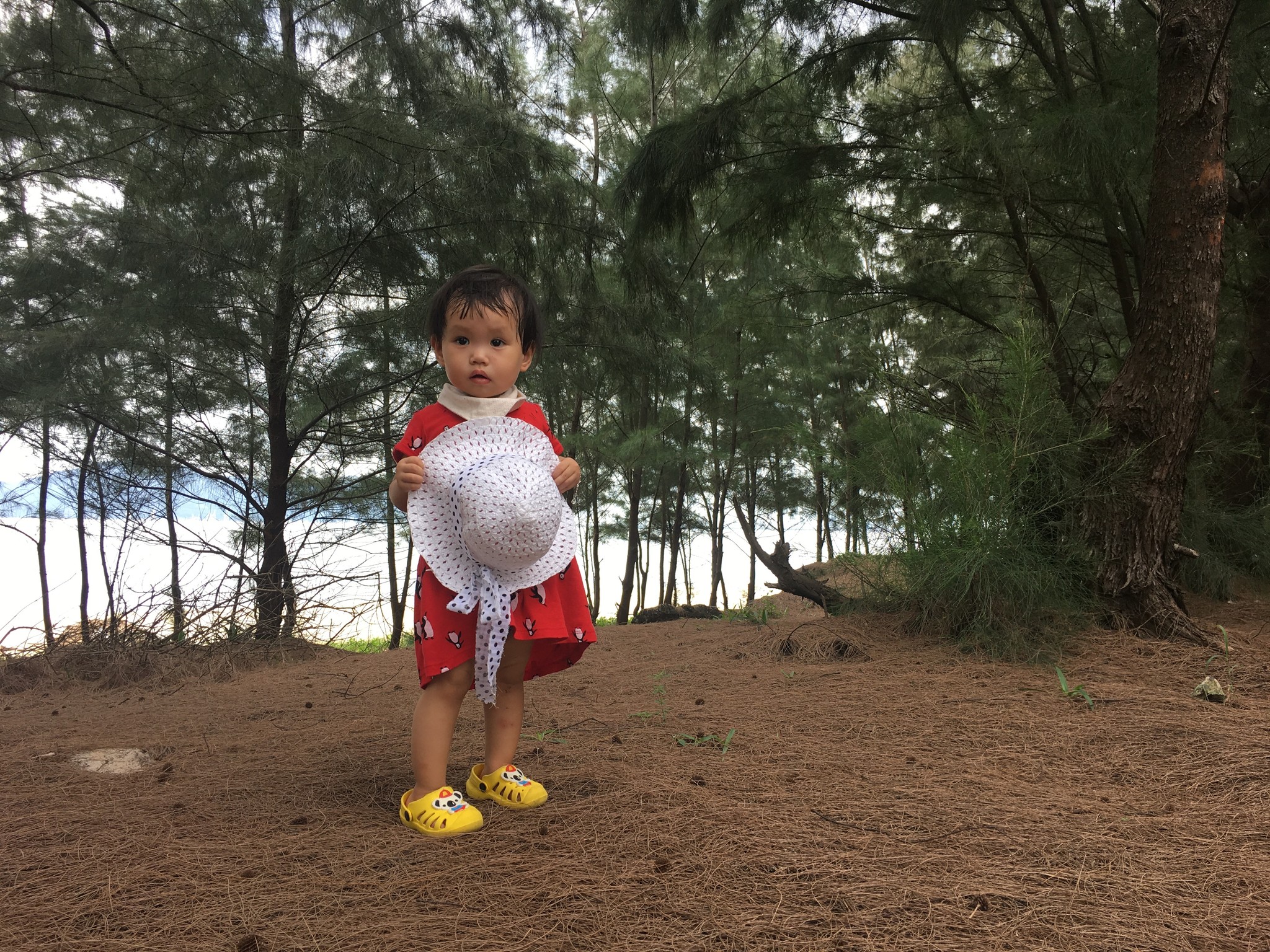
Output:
(399, 786), (484, 837)
(465, 763), (548, 809)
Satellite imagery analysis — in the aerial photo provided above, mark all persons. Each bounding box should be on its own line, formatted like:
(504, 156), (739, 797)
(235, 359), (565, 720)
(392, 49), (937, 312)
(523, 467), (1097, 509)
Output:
(387, 266), (596, 837)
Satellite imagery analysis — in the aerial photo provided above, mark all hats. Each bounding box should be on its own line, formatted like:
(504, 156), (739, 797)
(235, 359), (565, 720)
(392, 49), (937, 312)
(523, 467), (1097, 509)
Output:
(407, 416), (576, 706)
